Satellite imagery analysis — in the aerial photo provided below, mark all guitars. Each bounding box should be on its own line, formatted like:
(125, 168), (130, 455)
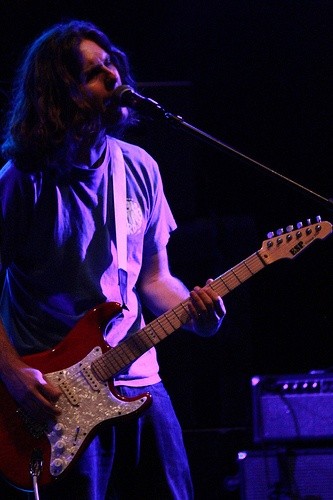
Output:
(0, 214), (333, 492)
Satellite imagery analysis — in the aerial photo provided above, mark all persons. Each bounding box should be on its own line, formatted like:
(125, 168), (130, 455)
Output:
(0, 21), (225, 500)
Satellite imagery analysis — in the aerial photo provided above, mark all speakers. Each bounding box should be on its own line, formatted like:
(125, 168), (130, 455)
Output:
(238, 449), (333, 500)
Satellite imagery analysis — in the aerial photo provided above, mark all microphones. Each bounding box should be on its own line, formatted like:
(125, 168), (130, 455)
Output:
(113, 85), (163, 112)
